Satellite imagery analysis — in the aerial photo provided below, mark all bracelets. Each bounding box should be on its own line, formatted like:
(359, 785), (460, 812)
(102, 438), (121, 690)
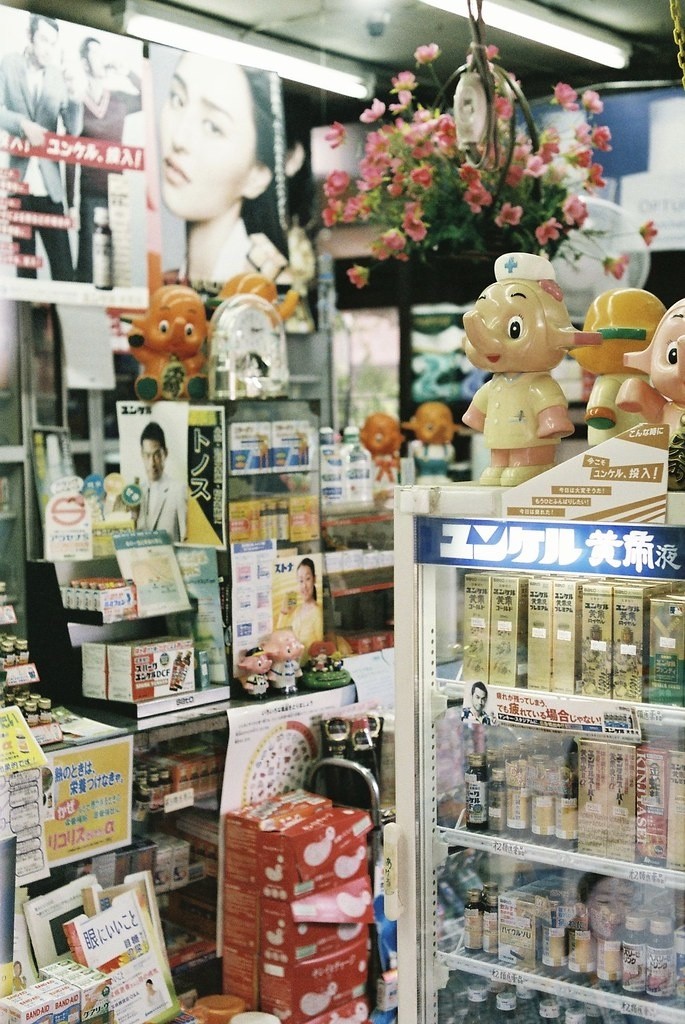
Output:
(281, 611), (287, 615)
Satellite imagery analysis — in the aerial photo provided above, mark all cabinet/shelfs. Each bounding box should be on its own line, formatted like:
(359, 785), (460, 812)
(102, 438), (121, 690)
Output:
(322, 512), (394, 659)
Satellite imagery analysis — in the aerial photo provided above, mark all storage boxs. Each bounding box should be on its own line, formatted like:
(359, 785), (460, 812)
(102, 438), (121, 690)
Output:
(106, 635), (195, 704)
(59, 583), (139, 623)
(81, 634), (147, 700)
(0, 745), (376, 1024)
(461, 569), (685, 971)
(227, 422), (320, 543)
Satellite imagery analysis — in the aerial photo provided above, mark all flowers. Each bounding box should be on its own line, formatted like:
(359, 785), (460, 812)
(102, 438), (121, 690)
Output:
(320, 34), (659, 290)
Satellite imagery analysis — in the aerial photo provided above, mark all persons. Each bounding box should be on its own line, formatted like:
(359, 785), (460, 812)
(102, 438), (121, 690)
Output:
(135, 423), (186, 542)
(0, 14), (143, 282)
(160, 52), (289, 283)
(461, 682), (490, 724)
(277, 558), (323, 648)
(564, 873), (634, 956)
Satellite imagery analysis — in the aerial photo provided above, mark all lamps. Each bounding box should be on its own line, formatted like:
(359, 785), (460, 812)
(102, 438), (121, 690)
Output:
(121, 0), (376, 101)
(419, 0), (633, 71)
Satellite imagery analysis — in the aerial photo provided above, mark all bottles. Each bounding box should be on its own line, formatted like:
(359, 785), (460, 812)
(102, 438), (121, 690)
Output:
(465, 735), (578, 849)
(438, 869), (685, 1024)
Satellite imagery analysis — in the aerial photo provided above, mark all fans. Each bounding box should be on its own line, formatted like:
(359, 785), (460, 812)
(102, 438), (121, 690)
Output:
(550, 194), (651, 324)
